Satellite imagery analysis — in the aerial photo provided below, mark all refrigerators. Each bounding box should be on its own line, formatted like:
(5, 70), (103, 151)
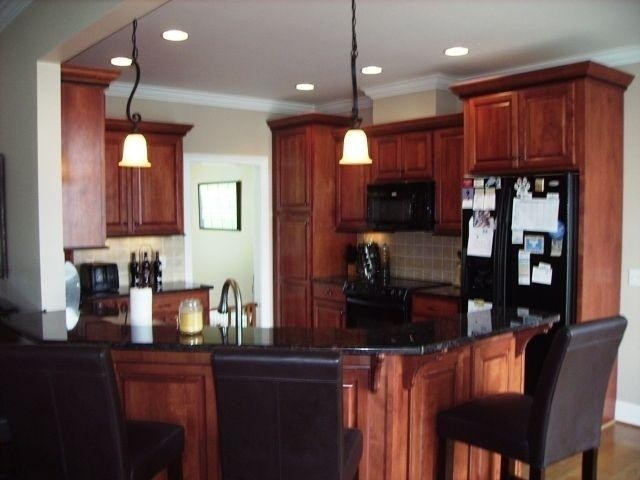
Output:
(463, 170), (576, 327)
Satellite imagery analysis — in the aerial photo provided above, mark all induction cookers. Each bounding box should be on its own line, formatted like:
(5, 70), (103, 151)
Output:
(343, 276), (451, 300)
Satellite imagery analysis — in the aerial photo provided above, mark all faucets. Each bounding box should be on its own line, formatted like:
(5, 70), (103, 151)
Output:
(218, 279), (242, 347)
(219, 326), (228, 346)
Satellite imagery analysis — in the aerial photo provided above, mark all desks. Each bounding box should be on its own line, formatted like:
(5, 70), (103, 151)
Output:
(0, 305), (566, 479)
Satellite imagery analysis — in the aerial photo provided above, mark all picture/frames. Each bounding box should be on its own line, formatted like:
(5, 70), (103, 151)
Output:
(198, 181), (242, 232)
(0, 153), (8, 278)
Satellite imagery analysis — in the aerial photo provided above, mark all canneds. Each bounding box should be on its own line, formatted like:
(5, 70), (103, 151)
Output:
(178, 297), (203, 336)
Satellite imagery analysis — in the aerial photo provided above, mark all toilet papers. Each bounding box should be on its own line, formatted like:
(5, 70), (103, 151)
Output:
(131, 325), (154, 344)
(130, 287), (153, 327)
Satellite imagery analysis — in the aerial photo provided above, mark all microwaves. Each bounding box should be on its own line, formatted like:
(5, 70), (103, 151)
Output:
(365, 180), (435, 230)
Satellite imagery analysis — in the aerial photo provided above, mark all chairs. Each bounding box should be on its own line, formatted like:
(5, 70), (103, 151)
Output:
(207, 342), (368, 479)
(428, 308), (630, 479)
(1, 340), (189, 479)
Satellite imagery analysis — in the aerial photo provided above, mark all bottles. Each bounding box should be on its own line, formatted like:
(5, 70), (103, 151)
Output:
(380, 244), (390, 272)
(154, 251), (163, 282)
(179, 297), (205, 334)
(142, 253), (150, 285)
(130, 251), (139, 284)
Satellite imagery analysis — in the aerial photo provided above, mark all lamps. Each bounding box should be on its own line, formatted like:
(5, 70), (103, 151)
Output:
(113, 15), (156, 170)
(334, 0), (374, 167)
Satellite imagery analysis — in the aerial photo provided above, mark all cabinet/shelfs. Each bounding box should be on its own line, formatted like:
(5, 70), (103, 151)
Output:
(264, 111), (362, 332)
(336, 124), (374, 234)
(104, 115), (196, 243)
(434, 111), (466, 240)
(370, 115), (433, 185)
(448, 58), (637, 175)
(410, 291), (461, 327)
(311, 279), (347, 330)
(61, 62), (122, 252)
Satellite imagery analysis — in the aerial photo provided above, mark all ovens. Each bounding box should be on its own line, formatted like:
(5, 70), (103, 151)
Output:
(345, 298), (408, 330)
